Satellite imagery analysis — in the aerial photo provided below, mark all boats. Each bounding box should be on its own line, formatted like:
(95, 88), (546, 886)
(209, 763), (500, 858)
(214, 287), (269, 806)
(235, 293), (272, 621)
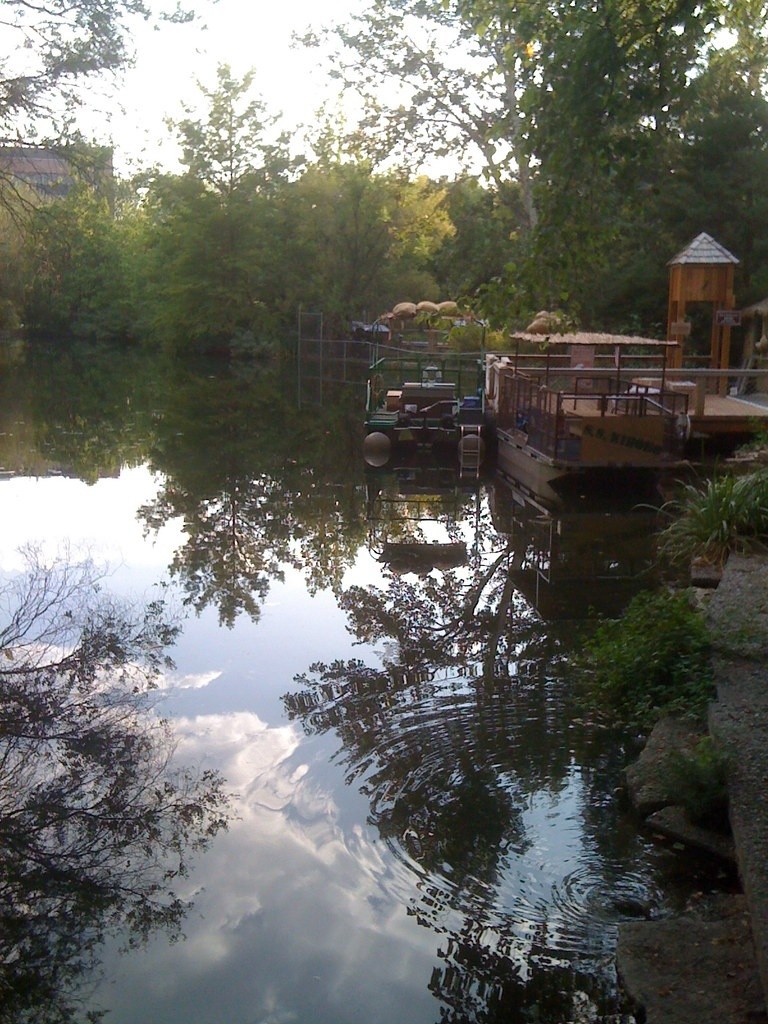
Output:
(362, 365), (487, 481)
